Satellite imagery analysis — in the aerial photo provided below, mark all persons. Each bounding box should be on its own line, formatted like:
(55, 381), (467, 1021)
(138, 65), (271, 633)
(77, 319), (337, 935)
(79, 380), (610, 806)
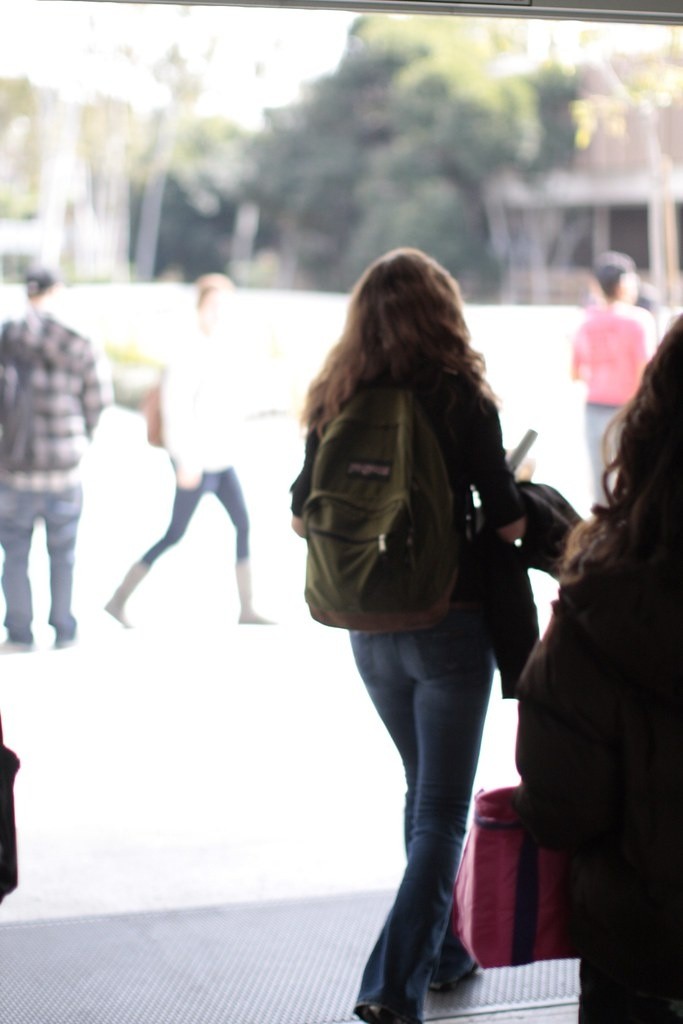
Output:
(0, 263), (110, 655)
(572, 253), (658, 506)
(105, 275), (278, 629)
(289, 247), (534, 1024)
(516, 316), (683, 1024)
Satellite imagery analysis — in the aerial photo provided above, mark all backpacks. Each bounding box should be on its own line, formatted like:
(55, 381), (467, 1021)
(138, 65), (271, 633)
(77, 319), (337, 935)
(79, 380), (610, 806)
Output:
(300, 391), (456, 633)
(143, 380), (162, 447)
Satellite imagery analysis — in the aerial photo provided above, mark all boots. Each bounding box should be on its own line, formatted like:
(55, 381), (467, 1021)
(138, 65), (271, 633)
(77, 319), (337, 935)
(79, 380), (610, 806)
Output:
(103, 560), (150, 629)
(236, 564), (280, 625)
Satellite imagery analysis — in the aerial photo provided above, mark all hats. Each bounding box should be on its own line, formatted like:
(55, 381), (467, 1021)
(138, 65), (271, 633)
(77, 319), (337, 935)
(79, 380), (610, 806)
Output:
(589, 252), (636, 283)
(27, 270), (55, 292)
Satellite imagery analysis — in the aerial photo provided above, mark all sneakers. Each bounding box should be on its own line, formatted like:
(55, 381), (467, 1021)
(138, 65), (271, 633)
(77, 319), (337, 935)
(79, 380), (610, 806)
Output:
(354, 1001), (425, 1024)
(429, 963), (478, 989)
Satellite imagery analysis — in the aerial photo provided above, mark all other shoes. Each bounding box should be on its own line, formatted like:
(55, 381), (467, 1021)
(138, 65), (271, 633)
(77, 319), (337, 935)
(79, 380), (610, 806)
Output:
(0, 641), (33, 653)
(56, 640), (77, 648)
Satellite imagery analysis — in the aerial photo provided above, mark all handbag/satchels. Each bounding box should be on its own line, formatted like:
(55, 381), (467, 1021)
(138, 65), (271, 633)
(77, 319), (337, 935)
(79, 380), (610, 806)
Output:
(1, 343), (37, 473)
(453, 784), (583, 968)
